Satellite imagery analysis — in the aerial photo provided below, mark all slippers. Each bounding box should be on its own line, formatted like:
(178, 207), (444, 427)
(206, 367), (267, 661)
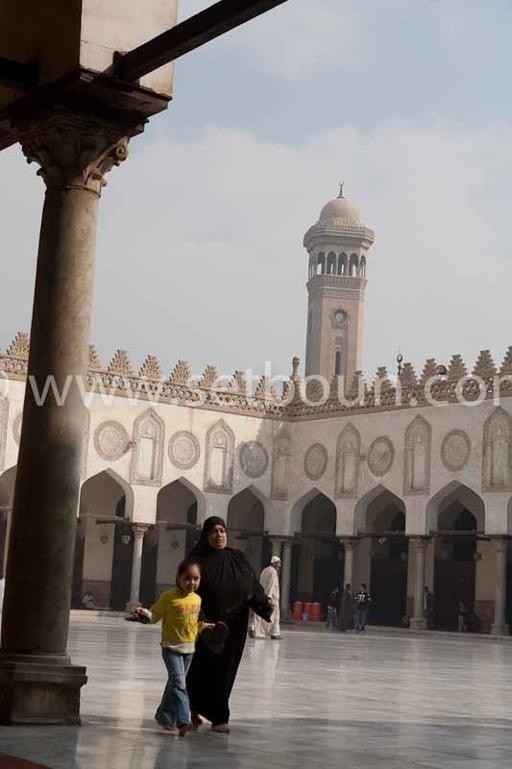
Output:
(125, 610), (151, 624)
(203, 622), (228, 653)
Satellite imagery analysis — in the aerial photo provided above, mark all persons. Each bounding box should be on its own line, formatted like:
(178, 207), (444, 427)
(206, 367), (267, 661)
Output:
(326, 583), (372, 633)
(423, 585), (434, 629)
(129, 515), (284, 738)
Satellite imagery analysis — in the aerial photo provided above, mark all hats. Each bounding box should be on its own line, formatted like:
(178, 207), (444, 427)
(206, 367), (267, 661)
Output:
(270, 556), (281, 564)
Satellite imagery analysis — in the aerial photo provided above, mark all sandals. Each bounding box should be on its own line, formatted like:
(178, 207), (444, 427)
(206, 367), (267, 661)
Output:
(247, 626), (256, 638)
(270, 635), (283, 640)
